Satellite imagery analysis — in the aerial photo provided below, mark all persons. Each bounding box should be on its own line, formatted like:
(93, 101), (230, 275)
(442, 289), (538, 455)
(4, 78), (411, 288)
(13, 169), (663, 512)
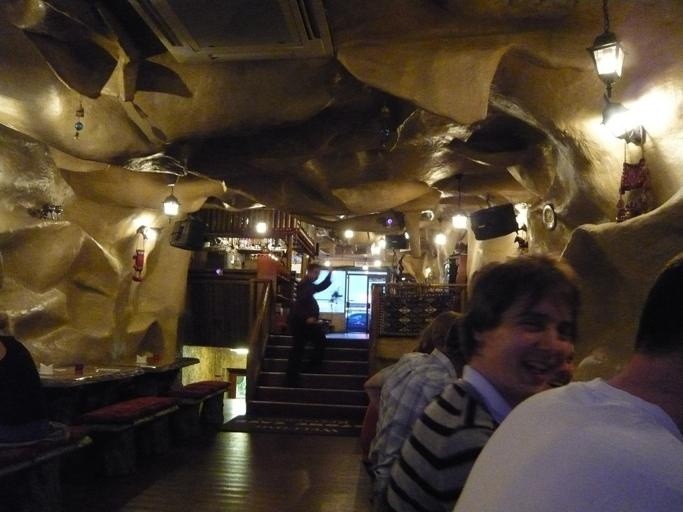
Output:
(385, 255), (580, 512)
(287, 261), (334, 373)
(362, 310), (467, 511)
(456, 253), (683, 511)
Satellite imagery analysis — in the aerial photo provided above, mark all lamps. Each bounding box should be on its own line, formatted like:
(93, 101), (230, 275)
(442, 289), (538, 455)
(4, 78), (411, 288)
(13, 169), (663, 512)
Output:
(451, 181), (467, 232)
(584, 0), (629, 128)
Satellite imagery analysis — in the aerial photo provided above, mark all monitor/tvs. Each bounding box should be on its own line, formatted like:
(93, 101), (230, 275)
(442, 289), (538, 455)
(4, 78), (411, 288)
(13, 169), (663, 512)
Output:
(386, 235), (409, 249)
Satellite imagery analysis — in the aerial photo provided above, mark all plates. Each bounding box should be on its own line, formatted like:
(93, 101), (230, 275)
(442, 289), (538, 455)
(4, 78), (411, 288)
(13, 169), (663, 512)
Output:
(542, 204), (557, 231)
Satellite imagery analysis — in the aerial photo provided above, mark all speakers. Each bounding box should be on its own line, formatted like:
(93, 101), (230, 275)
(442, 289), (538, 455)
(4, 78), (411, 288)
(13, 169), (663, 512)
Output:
(470, 203), (518, 240)
(169, 221), (206, 252)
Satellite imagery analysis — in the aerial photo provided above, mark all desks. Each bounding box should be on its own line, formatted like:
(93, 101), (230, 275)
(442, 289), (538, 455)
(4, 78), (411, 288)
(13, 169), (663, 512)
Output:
(32, 363), (144, 424)
(124, 355), (199, 394)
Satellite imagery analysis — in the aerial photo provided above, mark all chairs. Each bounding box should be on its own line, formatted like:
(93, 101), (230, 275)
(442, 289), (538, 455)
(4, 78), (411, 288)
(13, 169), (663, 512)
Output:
(0, 338), (94, 511)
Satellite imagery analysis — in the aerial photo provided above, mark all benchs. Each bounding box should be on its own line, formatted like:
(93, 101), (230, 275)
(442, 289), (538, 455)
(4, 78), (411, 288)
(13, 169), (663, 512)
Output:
(173, 380), (231, 445)
(80, 396), (176, 468)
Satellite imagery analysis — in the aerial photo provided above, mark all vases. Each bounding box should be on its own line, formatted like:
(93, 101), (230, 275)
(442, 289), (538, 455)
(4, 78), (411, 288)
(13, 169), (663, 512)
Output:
(161, 178), (181, 224)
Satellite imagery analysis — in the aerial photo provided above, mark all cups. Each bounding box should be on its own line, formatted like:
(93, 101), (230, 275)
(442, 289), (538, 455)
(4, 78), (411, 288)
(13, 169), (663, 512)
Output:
(218, 238), (285, 269)
(74, 361), (83, 376)
